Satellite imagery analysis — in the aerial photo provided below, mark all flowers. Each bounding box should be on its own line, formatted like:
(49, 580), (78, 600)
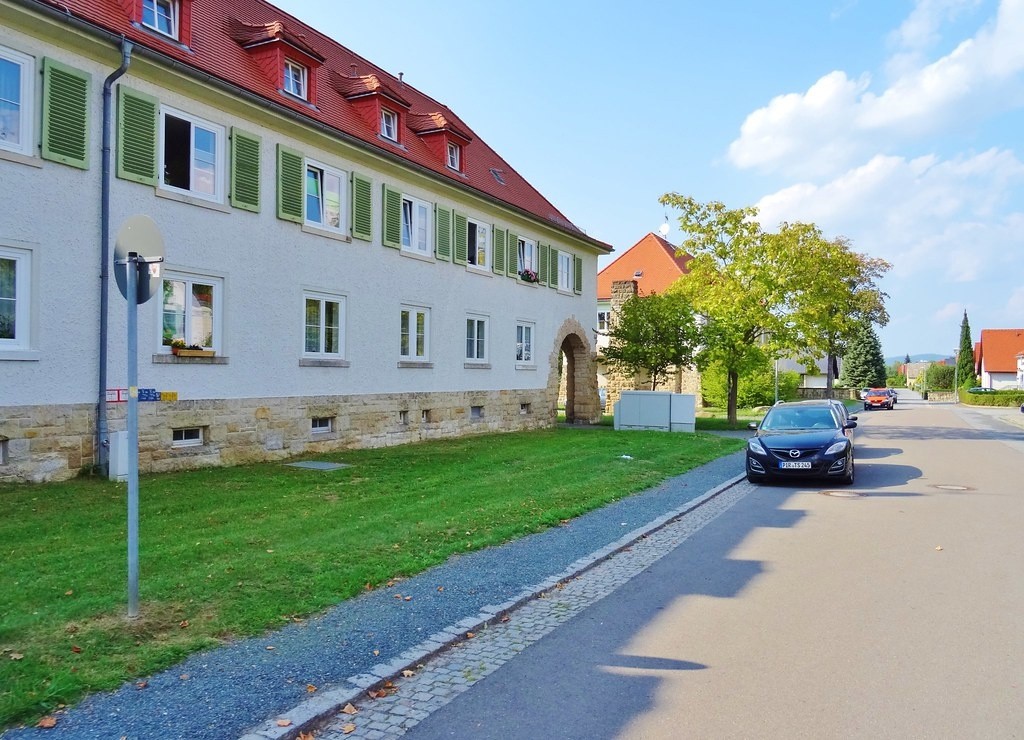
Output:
(171, 339), (203, 349)
(521, 269), (537, 283)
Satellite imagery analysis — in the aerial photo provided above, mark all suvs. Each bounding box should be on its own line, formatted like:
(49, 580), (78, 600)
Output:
(745, 397), (858, 485)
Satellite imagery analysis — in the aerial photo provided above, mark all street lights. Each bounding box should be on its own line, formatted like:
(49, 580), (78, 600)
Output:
(953, 347), (960, 404)
(919, 367), (927, 400)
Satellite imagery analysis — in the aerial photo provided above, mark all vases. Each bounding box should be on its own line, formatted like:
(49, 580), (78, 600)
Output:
(173, 349), (215, 357)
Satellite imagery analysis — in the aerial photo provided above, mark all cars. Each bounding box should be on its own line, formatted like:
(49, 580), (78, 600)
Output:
(860, 388), (872, 400)
(885, 388), (898, 403)
(803, 400), (858, 449)
(864, 388), (894, 411)
(969, 387), (996, 393)
